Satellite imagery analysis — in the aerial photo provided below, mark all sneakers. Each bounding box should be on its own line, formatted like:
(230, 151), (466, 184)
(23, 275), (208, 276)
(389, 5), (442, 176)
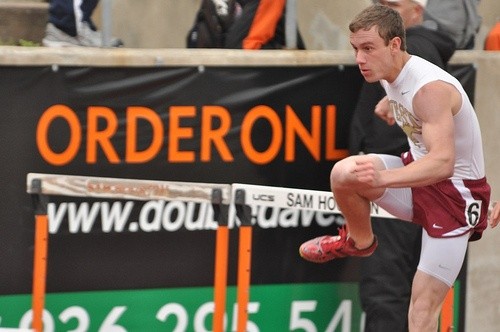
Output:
(42, 21), (123, 48)
(298, 223), (379, 262)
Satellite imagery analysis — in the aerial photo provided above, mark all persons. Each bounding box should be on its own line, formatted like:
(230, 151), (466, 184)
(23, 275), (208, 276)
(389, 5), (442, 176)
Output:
(345, 0), (458, 331)
(37, 0), (123, 48)
(186, 0), (306, 50)
(299, 4), (491, 331)
(415, 0), (483, 49)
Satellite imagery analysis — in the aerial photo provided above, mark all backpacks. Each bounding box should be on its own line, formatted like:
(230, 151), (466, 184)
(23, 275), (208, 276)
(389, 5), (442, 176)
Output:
(186, 0), (244, 49)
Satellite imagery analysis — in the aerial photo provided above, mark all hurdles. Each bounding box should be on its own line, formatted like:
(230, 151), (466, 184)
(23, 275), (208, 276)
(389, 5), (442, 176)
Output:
(22, 168), (232, 332)
(234, 175), (456, 332)
